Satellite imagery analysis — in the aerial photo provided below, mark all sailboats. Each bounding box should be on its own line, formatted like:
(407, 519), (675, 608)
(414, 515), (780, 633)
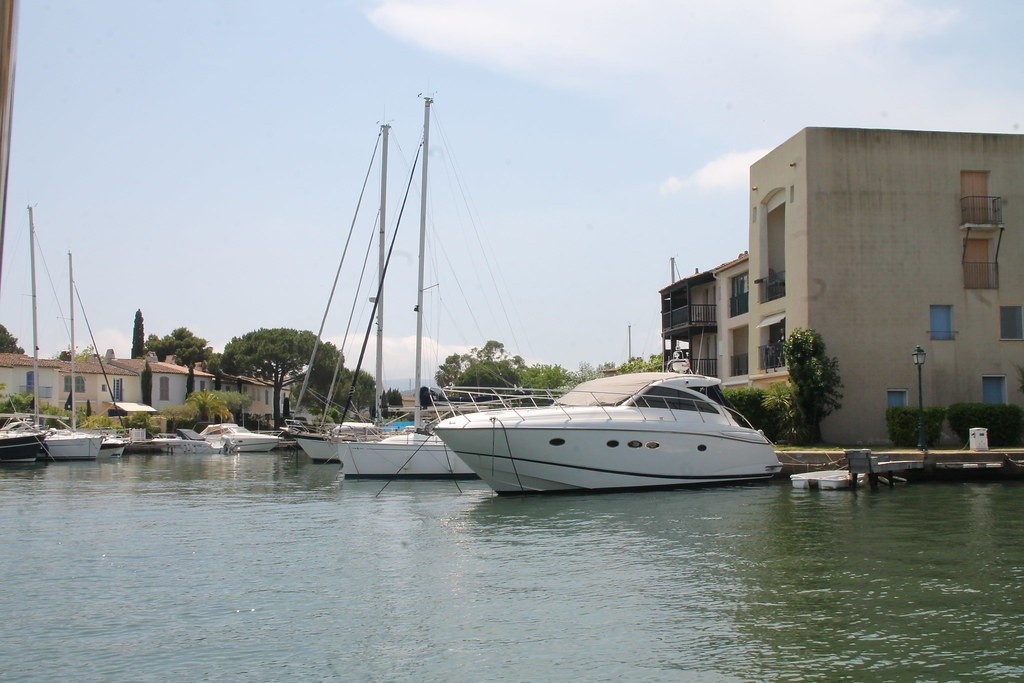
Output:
(0, 204), (131, 462)
(281, 95), (479, 481)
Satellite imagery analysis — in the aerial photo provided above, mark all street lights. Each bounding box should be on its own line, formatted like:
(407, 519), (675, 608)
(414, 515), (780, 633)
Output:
(912, 345), (928, 451)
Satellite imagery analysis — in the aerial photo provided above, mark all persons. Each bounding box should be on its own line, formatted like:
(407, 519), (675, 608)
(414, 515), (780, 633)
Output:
(764, 327), (785, 367)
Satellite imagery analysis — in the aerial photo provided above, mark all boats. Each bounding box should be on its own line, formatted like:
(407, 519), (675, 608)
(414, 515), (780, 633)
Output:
(790, 470), (871, 491)
(432, 350), (784, 496)
(153, 420), (282, 455)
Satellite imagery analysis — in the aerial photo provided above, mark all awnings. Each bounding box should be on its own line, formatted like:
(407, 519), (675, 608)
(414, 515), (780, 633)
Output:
(756, 311), (786, 328)
